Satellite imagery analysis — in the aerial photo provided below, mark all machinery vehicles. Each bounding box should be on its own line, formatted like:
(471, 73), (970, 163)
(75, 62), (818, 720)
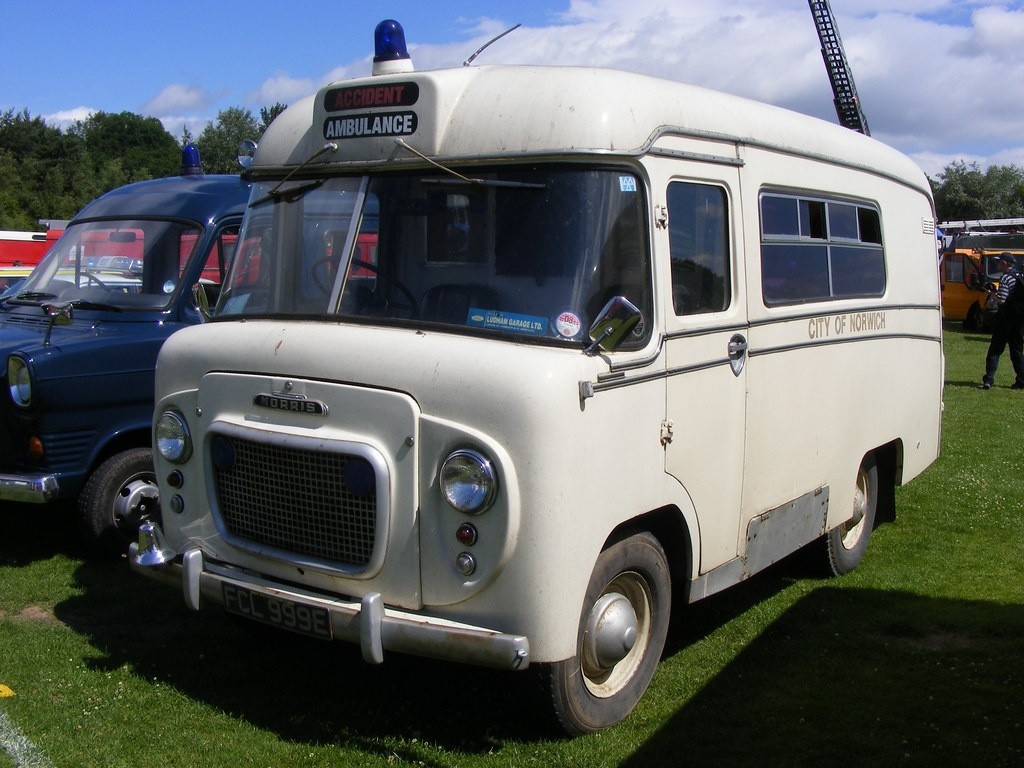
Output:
(807, 0), (873, 137)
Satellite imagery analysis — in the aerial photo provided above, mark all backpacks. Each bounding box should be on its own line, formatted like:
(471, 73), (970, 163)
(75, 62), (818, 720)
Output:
(1004, 272), (1024, 326)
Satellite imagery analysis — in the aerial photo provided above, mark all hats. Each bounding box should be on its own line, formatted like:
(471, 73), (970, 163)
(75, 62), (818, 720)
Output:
(994, 252), (1017, 266)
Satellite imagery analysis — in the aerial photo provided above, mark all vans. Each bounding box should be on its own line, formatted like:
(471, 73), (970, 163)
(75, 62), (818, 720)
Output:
(0, 143), (378, 558)
(116, 19), (946, 733)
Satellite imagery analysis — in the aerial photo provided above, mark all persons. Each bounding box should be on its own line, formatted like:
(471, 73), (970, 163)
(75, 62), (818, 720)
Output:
(982, 252), (1024, 389)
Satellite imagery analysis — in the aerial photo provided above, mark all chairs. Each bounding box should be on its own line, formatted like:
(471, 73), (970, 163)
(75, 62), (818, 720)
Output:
(417, 284), (497, 325)
(584, 284), (693, 341)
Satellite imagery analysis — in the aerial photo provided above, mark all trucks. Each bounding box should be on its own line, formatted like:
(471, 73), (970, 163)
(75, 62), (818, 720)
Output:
(938, 218), (1024, 330)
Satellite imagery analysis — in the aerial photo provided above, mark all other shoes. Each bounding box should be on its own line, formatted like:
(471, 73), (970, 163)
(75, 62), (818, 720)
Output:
(1012, 383), (1024, 389)
(982, 383), (990, 389)
